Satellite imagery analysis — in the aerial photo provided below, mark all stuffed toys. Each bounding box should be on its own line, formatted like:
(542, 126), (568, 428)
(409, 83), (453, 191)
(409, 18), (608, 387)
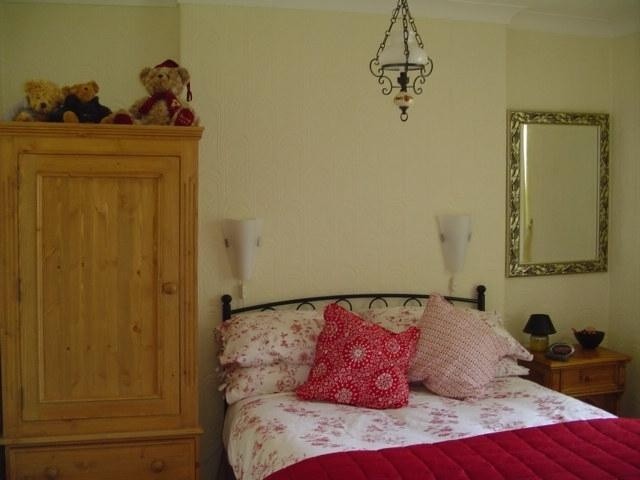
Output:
(14, 80), (61, 122)
(61, 80), (113, 124)
(114, 60), (199, 126)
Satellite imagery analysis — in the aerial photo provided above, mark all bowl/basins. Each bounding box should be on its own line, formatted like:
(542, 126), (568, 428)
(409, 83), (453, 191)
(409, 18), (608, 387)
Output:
(575, 330), (604, 350)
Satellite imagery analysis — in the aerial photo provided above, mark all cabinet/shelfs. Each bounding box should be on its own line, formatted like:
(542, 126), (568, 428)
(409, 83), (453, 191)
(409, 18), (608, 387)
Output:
(0, 121), (205, 480)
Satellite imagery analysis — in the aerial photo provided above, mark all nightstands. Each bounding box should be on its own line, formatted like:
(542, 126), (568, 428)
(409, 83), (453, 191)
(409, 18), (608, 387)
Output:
(516, 343), (632, 417)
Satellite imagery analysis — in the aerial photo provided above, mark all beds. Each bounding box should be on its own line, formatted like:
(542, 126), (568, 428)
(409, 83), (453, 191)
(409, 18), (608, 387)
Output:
(214, 284), (640, 480)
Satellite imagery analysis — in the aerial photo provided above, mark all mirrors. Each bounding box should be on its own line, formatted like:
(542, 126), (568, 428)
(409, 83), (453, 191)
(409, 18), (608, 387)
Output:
(505, 109), (610, 278)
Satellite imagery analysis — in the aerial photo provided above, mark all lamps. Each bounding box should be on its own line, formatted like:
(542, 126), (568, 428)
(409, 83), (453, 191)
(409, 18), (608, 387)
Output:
(370, 0), (433, 121)
(523, 314), (557, 352)
(437, 214), (475, 293)
(221, 217), (260, 298)
